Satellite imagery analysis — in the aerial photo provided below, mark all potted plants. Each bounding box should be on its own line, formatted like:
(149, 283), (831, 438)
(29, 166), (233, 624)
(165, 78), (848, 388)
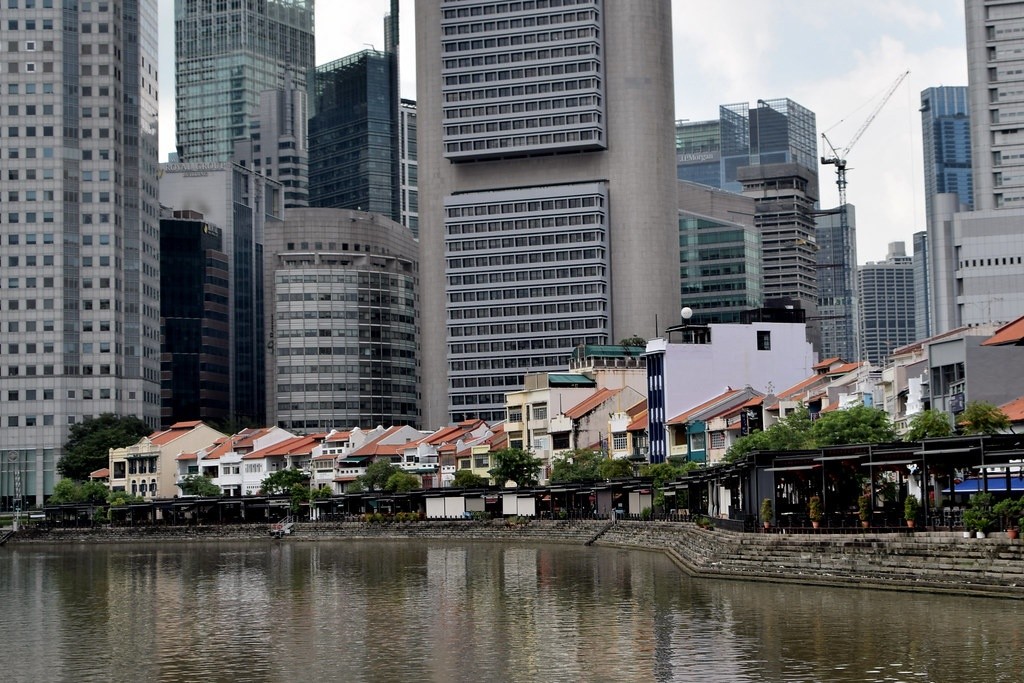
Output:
(904, 495), (919, 528)
(961, 493), (995, 538)
(761, 497), (773, 528)
(857, 495), (872, 527)
(694, 516), (716, 531)
(994, 497), (1024, 539)
(810, 496), (823, 528)
